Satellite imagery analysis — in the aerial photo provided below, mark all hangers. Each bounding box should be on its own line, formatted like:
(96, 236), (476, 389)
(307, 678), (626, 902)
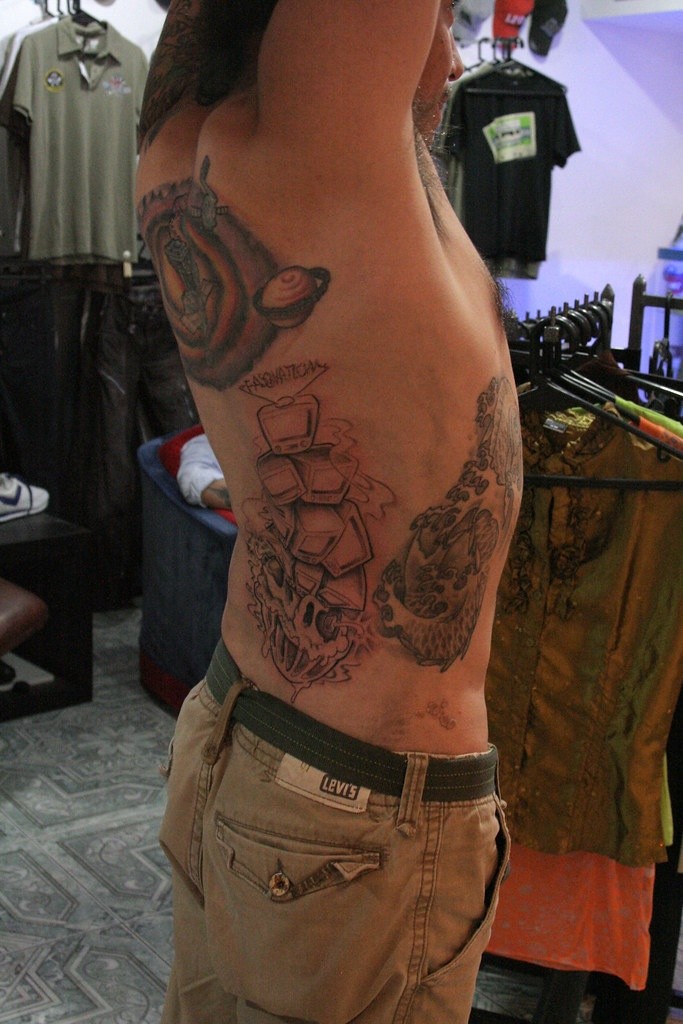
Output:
(462, 36), (568, 93)
(508, 288), (683, 495)
(44, 0), (105, 30)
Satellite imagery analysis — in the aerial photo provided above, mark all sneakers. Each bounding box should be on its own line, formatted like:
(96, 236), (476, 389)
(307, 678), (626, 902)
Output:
(0, 474), (49, 522)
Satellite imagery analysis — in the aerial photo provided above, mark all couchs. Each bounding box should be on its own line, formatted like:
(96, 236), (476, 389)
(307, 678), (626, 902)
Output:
(135, 421), (241, 721)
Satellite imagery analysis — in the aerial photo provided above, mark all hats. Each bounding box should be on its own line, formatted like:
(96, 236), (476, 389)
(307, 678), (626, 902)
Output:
(493, 0), (535, 41)
(529, 0), (567, 55)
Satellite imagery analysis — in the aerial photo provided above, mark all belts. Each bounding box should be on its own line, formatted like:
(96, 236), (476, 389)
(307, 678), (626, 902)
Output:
(202, 637), (497, 802)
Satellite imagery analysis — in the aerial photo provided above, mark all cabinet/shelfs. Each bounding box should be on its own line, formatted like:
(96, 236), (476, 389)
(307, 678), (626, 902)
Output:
(0, 514), (96, 721)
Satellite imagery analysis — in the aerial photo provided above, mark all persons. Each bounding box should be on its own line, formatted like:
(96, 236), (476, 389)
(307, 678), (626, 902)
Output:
(131, 0), (528, 1024)
